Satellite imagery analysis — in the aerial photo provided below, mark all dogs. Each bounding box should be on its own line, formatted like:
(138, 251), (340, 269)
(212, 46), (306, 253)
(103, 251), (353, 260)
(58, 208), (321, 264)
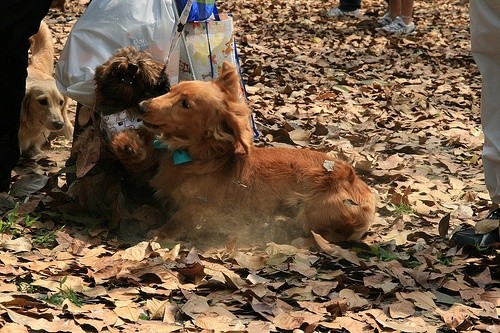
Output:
(111, 61), (378, 248)
(18, 21), (72, 158)
(71, 46), (171, 229)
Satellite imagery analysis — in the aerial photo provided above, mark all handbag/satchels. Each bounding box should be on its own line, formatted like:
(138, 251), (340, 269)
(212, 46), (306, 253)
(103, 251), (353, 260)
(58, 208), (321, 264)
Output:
(181, 13), (259, 138)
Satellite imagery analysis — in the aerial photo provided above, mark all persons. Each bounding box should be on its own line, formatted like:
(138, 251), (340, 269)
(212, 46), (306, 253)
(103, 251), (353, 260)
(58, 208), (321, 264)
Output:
(328, 0), (361, 18)
(470, 0), (500, 247)
(374, 0), (415, 34)
(0, 0), (66, 194)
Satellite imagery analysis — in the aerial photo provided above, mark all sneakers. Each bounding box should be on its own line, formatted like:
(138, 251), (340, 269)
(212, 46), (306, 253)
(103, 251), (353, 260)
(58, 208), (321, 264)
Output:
(455, 208), (500, 248)
(382, 16), (415, 33)
(326, 6), (360, 16)
(378, 12), (392, 25)
